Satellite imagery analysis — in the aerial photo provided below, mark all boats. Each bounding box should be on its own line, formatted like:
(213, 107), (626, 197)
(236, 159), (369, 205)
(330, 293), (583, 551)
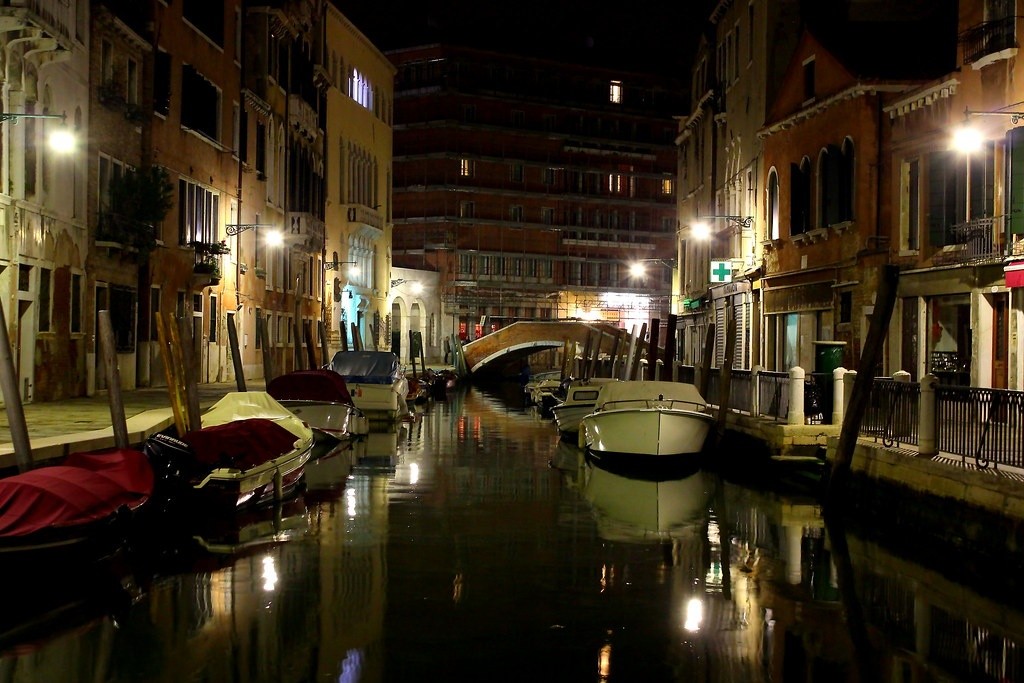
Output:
(576, 452), (716, 545)
(354, 429), (400, 460)
(168, 390), (316, 508)
(302, 439), (356, 492)
(405, 369), (459, 401)
(0, 447), (155, 558)
(576, 377), (719, 458)
(548, 377), (625, 434)
(263, 368), (373, 439)
(322, 348), (413, 424)
(183, 495), (313, 556)
(523, 371), (563, 409)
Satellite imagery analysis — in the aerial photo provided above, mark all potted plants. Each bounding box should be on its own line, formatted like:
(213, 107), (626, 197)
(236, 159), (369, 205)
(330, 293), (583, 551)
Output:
(193, 262), (214, 284)
(210, 257), (221, 285)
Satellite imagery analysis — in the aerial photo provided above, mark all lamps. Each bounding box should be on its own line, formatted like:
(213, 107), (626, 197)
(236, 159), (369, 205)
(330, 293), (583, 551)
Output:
(334, 277), (353, 301)
